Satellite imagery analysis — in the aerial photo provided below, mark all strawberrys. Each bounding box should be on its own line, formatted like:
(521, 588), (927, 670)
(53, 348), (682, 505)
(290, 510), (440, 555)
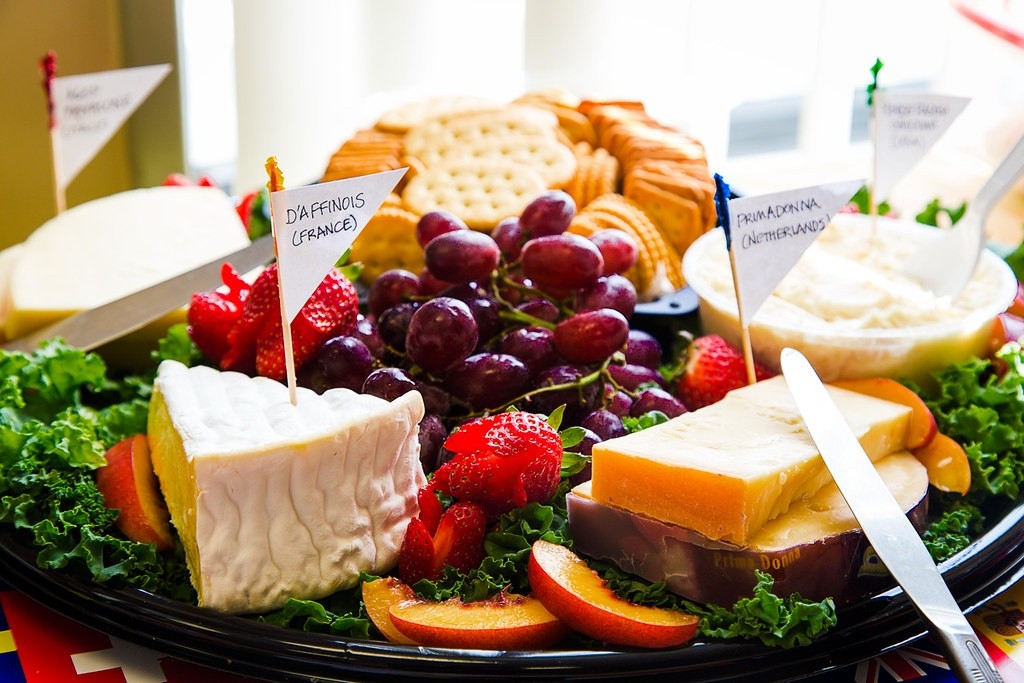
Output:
(680, 335), (770, 412)
(400, 487), (487, 583)
(159, 172), (362, 380)
(429, 413), (563, 505)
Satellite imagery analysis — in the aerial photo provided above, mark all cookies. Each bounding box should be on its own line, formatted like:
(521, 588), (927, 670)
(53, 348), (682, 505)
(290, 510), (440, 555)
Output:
(321, 89), (719, 301)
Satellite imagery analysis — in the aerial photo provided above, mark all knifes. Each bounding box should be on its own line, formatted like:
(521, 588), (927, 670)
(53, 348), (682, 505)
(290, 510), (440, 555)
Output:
(1, 234), (276, 352)
(781, 347), (1005, 681)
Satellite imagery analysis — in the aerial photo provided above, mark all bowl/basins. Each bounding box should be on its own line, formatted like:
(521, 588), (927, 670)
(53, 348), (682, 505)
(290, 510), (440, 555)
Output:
(681, 215), (1020, 384)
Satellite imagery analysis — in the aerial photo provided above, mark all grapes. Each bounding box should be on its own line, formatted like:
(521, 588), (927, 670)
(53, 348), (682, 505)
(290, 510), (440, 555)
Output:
(301, 186), (684, 484)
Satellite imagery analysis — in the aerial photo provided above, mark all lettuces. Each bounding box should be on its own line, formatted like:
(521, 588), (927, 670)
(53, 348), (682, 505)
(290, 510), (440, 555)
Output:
(0, 321), (1024, 648)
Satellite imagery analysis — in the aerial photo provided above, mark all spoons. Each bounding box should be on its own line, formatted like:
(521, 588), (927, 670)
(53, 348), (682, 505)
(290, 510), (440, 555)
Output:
(891, 129), (1021, 312)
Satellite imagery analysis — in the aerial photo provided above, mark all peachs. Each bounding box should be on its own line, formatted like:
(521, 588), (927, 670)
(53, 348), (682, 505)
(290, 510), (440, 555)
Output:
(917, 435), (971, 496)
(390, 592), (561, 650)
(362, 576), (420, 645)
(831, 378), (936, 449)
(528, 539), (699, 648)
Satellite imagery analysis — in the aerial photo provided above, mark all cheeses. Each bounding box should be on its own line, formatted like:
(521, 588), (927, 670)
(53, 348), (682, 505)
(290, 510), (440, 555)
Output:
(706, 212), (1003, 377)
(565, 452), (929, 611)
(147, 359), (426, 611)
(592, 375), (913, 545)
(0, 185), (259, 340)
